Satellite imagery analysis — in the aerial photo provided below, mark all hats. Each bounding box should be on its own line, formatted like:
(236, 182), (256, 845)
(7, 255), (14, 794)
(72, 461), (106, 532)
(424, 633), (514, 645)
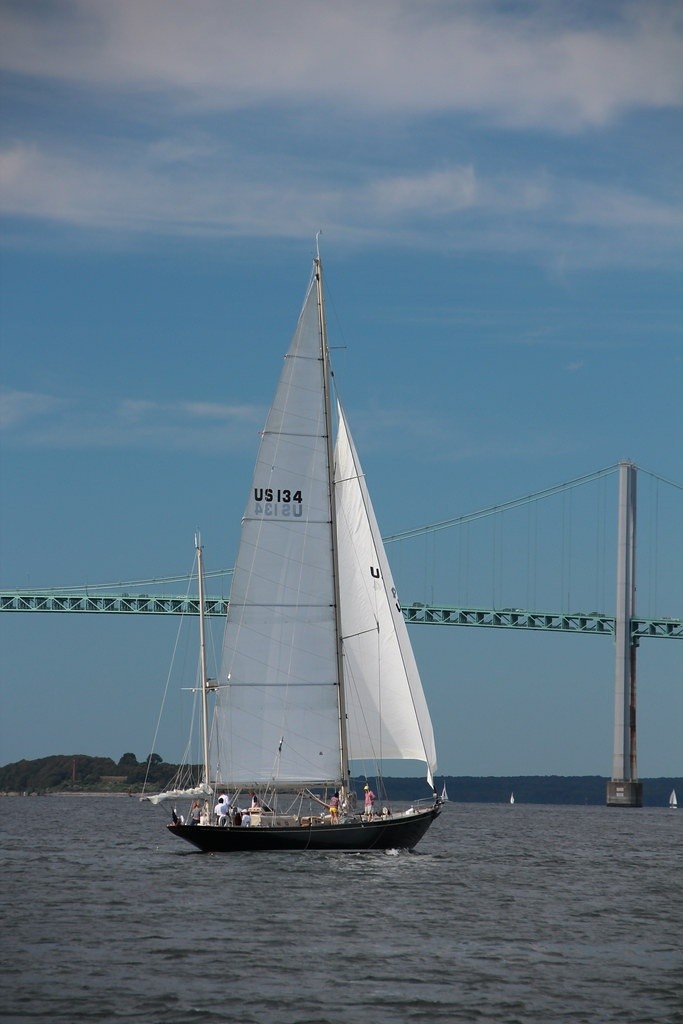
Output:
(241, 809), (248, 813)
(364, 786), (369, 790)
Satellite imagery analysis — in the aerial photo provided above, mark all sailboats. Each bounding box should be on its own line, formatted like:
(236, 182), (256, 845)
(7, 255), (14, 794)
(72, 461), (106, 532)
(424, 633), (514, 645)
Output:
(136, 230), (451, 851)
(668, 789), (679, 808)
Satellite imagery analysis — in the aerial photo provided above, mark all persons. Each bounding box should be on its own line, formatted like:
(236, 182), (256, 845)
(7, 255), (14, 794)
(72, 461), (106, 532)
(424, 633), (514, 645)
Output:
(329, 792), (343, 825)
(249, 791), (258, 808)
(189, 801), (200, 826)
(232, 809), (251, 827)
(362, 785), (376, 822)
(214, 789), (230, 827)
